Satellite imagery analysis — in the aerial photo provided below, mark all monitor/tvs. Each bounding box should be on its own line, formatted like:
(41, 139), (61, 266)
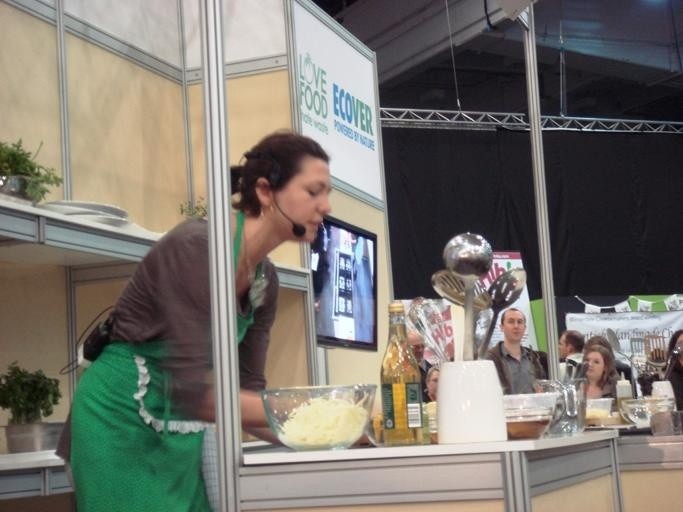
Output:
(312, 213), (377, 352)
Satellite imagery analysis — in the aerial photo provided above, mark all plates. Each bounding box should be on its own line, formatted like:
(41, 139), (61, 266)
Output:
(39, 200), (129, 227)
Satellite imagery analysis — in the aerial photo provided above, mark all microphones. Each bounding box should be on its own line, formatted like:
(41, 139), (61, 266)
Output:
(273, 182), (305, 237)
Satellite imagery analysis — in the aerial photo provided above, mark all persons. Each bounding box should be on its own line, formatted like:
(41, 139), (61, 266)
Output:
(69, 129), (332, 511)
(409, 306), (683, 411)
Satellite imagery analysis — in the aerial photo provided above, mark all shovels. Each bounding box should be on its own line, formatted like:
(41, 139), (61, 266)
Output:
(432, 269), (495, 360)
(631, 335), (668, 381)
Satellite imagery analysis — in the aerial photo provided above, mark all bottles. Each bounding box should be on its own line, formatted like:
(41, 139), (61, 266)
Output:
(377, 302), (430, 446)
(616, 372), (634, 424)
(437, 359), (507, 442)
(651, 381), (676, 413)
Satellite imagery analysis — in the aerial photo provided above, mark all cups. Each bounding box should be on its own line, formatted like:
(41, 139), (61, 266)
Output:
(637, 371), (658, 394)
(528, 378), (588, 438)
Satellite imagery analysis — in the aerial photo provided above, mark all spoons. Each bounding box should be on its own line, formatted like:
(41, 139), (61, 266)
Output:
(605, 327), (642, 377)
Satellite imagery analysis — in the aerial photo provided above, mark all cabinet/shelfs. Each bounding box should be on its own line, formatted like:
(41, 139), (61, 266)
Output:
(0, 192), (314, 508)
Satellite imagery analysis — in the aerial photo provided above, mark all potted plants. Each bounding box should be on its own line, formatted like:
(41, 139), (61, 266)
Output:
(0, 360), (64, 454)
(0, 134), (65, 207)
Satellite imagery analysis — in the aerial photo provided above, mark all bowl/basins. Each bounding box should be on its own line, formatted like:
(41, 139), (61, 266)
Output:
(1, 422), (65, 454)
(501, 392), (559, 439)
(586, 395), (675, 429)
(0, 176), (39, 207)
(257, 384), (376, 450)
